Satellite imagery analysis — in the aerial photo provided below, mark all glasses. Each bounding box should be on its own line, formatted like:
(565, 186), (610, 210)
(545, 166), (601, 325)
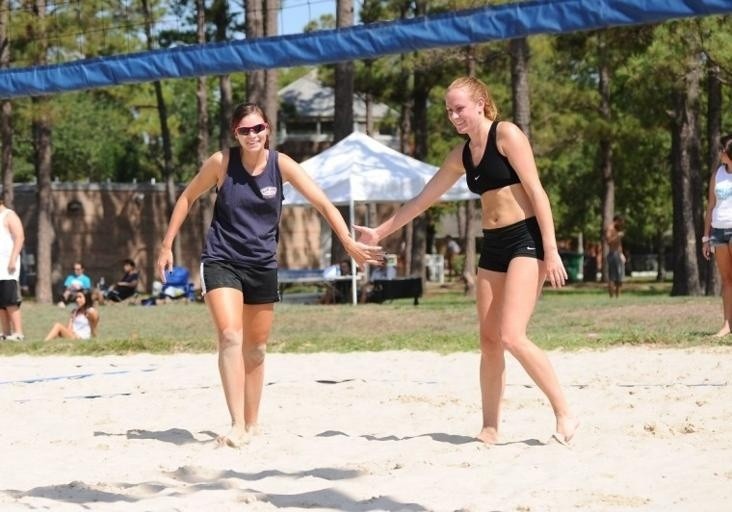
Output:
(238, 123), (267, 135)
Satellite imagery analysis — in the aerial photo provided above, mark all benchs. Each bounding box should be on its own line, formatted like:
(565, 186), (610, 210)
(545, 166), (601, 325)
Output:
(275, 268), (423, 305)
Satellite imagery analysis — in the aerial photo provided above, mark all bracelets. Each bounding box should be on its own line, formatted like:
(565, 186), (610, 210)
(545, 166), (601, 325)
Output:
(702, 236), (709, 242)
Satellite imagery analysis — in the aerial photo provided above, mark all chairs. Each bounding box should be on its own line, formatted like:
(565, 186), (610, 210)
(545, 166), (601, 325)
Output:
(156, 267), (195, 301)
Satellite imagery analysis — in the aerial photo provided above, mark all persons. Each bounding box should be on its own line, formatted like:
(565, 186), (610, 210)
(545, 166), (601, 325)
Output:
(45, 258), (139, 341)
(350, 75), (578, 447)
(443, 234), (462, 282)
(604, 214), (627, 299)
(0, 199), (25, 343)
(700, 135), (732, 339)
(157, 102), (387, 445)
(316, 260), (352, 304)
(358, 252), (397, 304)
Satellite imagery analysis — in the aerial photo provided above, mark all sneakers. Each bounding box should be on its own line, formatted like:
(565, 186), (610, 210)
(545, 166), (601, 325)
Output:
(0, 332), (23, 341)
